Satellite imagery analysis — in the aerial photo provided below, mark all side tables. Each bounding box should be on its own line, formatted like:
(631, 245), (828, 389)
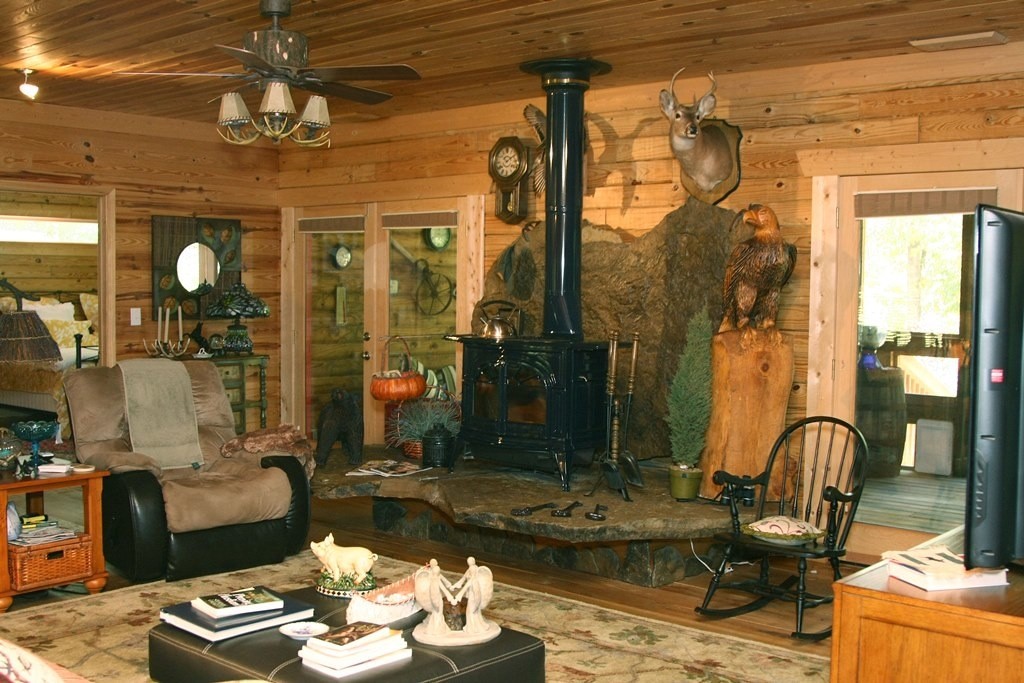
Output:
(0, 461), (113, 614)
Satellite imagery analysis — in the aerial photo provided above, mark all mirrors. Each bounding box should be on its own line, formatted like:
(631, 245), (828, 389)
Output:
(150, 212), (245, 323)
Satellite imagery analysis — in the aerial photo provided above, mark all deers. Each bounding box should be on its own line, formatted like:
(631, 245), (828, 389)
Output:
(660, 66), (733, 191)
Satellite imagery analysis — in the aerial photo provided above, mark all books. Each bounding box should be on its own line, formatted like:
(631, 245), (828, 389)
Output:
(298, 621), (412, 679)
(158, 585), (317, 642)
(881, 544), (1010, 592)
(345, 459), (434, 478)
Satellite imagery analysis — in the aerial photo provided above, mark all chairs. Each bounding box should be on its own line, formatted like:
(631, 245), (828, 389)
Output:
(692, 410), (872, 639)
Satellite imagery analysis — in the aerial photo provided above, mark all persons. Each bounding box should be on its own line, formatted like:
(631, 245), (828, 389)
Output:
(415, 556), (494, 636)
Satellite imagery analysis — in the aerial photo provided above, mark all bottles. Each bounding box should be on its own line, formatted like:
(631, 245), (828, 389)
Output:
(422, 423), (454, 468)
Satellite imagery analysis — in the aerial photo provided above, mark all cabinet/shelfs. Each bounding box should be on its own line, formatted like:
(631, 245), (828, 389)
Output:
(827, 522), (1024, 683)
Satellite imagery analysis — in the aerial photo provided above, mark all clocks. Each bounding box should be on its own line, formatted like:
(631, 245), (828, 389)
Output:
(486, 134), (531, 226)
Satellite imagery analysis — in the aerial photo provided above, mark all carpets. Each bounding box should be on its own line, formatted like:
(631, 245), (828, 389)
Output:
(1, 550), (830, 683)
(852, 462), (966, 533)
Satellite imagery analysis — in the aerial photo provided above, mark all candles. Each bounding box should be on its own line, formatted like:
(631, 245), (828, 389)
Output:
(163, 306), (171, 343)
(177, 304), (184, 341)
(157, 306), (162, 342)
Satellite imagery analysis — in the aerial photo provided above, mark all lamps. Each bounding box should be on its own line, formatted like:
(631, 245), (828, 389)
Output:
(205, 281), (271, 355)
(0, 276), (64, 366)
(14, 67), (41, 101)
(213, 77), (333, 151)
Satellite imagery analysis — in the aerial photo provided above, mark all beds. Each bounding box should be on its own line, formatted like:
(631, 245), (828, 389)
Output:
(0, 287), (99, 414)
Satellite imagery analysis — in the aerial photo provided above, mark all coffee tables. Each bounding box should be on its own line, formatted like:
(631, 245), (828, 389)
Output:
(146, 562), (547, 683)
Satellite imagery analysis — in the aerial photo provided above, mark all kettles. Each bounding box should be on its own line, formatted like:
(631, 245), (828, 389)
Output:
(480, 299), (517, 338)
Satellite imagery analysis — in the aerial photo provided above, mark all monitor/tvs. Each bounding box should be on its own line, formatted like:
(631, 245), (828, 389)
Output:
(962, 203), (1024, 572)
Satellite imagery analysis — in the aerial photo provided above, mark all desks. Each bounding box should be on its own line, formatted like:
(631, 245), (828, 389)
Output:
(149, 350), (270, 435)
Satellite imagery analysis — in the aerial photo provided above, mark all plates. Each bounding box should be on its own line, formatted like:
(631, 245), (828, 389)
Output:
(279, 621), (331, 640)
(192, 354), (213, 359)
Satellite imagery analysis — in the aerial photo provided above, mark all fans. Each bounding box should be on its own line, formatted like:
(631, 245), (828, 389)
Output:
(109, 0), (423, 106)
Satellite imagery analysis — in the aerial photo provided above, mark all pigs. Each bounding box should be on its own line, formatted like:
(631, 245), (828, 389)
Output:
(310, 533), (378, 585)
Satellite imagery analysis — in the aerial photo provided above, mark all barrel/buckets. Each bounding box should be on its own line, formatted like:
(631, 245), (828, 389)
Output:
(855, 367), (906, 478)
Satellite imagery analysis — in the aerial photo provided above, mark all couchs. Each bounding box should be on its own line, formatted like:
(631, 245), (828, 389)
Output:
(64, 358), (318, 588)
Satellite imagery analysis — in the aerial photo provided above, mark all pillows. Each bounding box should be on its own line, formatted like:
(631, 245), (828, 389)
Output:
(0, 294), (60, 316)
(22, 300), (76, 321)
(41, 319), (98, 347)
(79, 291), (99, 336)
